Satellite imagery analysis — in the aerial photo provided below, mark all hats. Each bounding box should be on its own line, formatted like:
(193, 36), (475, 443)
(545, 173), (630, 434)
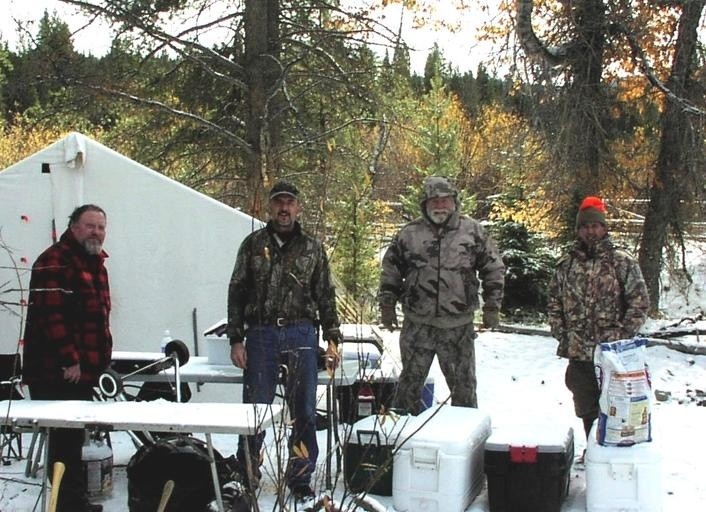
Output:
(576, 196), (608, 232)
(268, 180), (299, 201)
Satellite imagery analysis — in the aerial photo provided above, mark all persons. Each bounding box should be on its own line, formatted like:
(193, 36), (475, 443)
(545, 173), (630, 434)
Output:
(220, 180), (346, 504)
(376, 173), (505, 417)
(18, 204), (113, 511)
(544, 195), (649, 473)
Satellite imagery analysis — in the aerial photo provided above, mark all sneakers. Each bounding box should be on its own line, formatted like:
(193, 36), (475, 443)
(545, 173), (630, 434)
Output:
(288, 480), (317, 503)
(55, 501), (103, 512)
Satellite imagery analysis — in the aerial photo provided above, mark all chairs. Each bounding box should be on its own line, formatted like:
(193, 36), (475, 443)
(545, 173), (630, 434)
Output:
(0, 350), (27, 464)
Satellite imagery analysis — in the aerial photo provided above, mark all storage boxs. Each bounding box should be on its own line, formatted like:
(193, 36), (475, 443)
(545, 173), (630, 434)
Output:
(390, 404), (490, 511)
(585, 417), (658, 511)
(342, 414), (413, 496)
(336, 324), (434, 424)
(202, 317), (246, 369)
(484, 420), (574, 512)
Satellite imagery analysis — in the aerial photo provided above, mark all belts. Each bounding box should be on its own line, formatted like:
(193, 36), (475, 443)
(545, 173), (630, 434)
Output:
(248, 316), (316, 327)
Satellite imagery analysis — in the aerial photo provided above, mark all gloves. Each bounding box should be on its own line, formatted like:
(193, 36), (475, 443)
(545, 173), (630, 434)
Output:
(378, 306), (401, 332)
(482, 308), (501, 331)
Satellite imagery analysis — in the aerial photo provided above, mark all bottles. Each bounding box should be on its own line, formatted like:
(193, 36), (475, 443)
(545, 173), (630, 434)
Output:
(160, 329), (172, 358)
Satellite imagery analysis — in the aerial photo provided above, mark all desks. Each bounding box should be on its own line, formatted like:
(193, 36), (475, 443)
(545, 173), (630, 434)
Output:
(23, 353), (358, 491)
(1, 398), (285, 511)
